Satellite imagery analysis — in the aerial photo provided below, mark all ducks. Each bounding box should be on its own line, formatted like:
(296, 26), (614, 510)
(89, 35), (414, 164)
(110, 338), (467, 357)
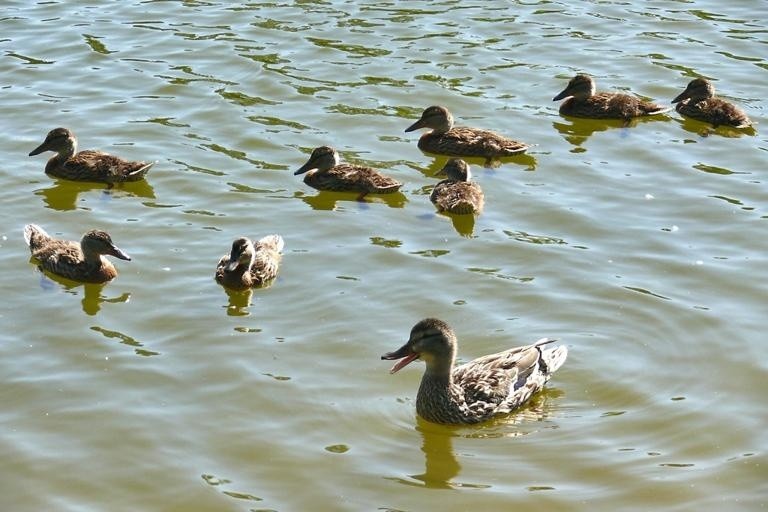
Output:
(215, 234), (285, 288)
(28, 127), (159, 200)
(23, 223), (132, 291)
(416, 158), (485, 220)
(405, 386), (565, 483)
(380, 317), (569, 426)
(404, 105), (540, 176)
(293, 145), (403, 210)
(552, 73), (675, 138)
(670, 77), (759, 142)
